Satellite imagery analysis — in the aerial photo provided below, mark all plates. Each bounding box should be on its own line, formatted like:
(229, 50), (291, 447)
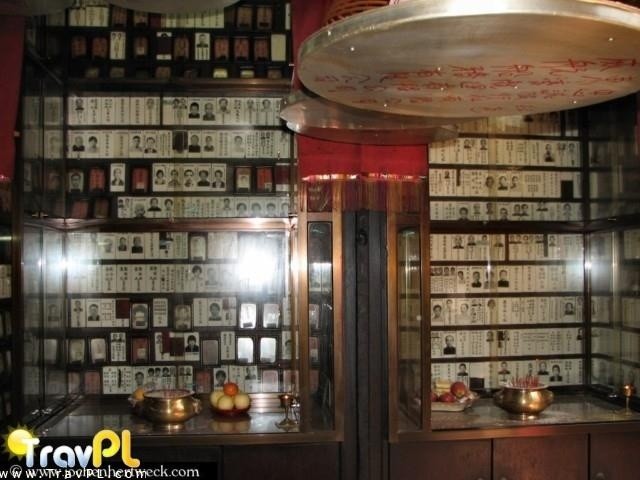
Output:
(208, 405), (251, 416)
(415, 399), (467, 413)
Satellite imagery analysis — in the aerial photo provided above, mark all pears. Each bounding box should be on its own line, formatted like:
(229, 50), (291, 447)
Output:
(210, 391), (249, 410)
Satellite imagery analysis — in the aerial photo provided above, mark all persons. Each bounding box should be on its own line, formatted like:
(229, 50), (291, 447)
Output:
(23, 5), (292, 395)
(598, 362), (640, 394)
(433, 137), (584, 382)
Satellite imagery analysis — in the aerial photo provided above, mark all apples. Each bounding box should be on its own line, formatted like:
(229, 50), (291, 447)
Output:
(134, 389), (145, 400)
(224, 383), (238, 396)
(431, 382), (466, 402)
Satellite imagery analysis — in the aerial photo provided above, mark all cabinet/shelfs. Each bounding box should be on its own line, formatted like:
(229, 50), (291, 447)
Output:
(0, 14), (640, 480)
